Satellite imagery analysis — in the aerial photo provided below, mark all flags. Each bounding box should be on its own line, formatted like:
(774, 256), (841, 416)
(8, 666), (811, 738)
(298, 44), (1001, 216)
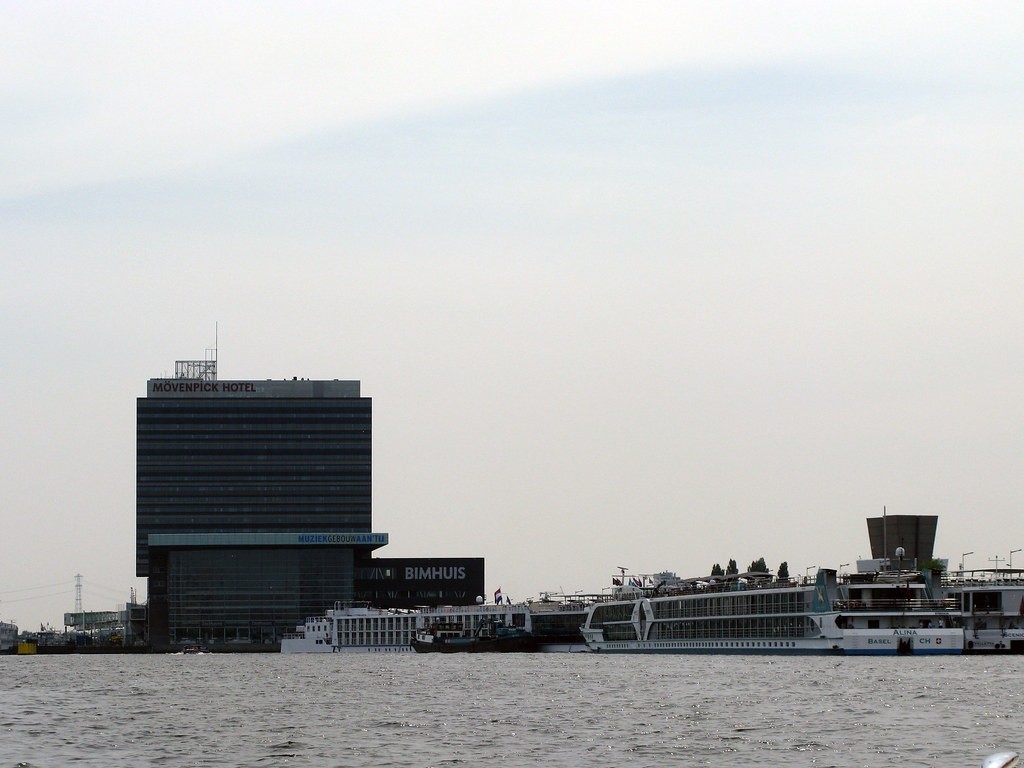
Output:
(495, 589), (502, 605)
(613, 578), (654, 588)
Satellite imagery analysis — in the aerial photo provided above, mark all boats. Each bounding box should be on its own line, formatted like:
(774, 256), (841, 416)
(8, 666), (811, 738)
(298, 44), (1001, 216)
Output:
(182, 644), (210, 654)
(409, 615), (549, 654)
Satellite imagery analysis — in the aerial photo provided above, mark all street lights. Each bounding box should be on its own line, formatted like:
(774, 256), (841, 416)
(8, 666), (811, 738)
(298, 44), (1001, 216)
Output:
(574, 590), (584, 600)
(988, 555), (1006, 586)
(806, 565), (816, 577)
(1009, 548), (1022, 586)
(602, 587), (610, 603)
(962, 552), (973, 576)
(840, 564), (850, 583)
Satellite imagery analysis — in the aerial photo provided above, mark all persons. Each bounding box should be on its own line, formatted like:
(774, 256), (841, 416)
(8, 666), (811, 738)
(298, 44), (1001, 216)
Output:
(938, 618), (944, 628)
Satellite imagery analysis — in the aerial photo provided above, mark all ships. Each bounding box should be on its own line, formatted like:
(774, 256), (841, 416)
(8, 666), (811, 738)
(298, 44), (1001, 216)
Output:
(578, 548), (1024, 654)
